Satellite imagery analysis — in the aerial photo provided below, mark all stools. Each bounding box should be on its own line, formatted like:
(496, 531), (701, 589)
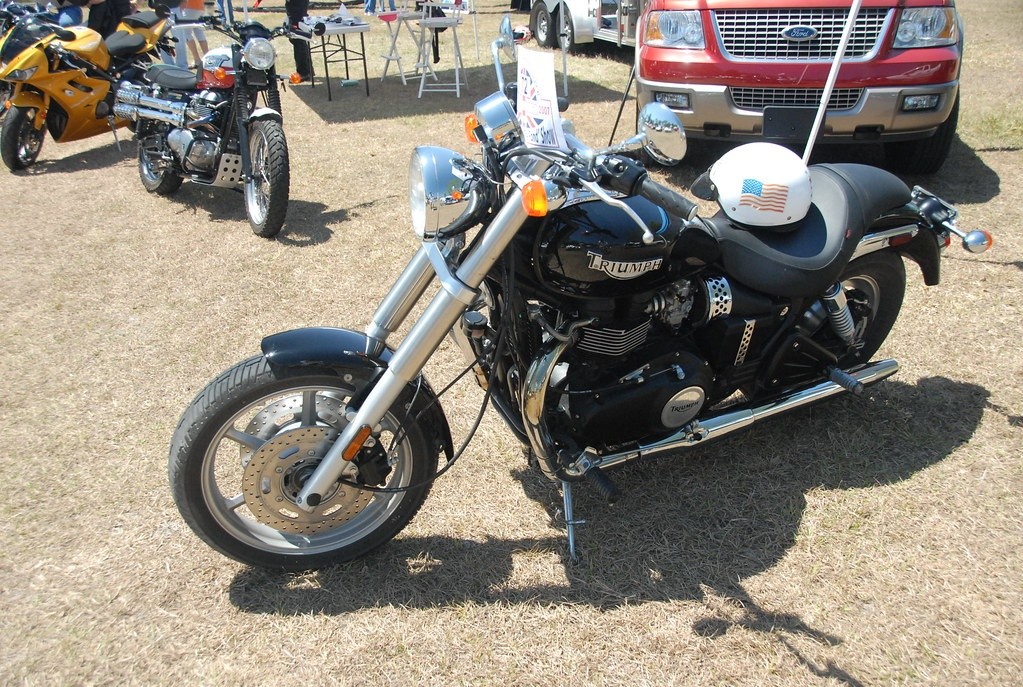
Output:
(378, 0), (470, 98)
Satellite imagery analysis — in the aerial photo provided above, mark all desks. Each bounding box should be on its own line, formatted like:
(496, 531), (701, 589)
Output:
(298, 20), (370, 101)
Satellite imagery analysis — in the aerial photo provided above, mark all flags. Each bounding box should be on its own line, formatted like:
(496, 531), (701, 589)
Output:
(738, 179), (790, 213)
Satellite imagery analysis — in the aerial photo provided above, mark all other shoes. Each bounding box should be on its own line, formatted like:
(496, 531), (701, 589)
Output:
(365, 12), (377, 16)
(289, 74), (310, 83)
(188, 62), (199, 69)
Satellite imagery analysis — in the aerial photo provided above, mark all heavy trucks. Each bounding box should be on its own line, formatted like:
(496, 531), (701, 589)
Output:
(512, 0), (966, 178)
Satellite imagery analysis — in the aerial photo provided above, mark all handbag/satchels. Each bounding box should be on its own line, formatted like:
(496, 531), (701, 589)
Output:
(415, 0), (448, 64)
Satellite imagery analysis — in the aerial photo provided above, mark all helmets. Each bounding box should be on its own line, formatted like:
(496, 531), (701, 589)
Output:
(690, 142), (813, 233)
(201, 46), (233, 73)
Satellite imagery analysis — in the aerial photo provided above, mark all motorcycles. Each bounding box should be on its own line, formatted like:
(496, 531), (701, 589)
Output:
(0, 0), (179, 171)
(166, 13), (992, 575)
(111, 3), (326, 239)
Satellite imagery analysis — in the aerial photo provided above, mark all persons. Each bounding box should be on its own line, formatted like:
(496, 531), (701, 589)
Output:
(57, 0), (142, 42)
(217, 0), (235, 25)
(285, 0), (315, 83)
(160, 0), (210, 69)
(364, 0), (397, 14)
